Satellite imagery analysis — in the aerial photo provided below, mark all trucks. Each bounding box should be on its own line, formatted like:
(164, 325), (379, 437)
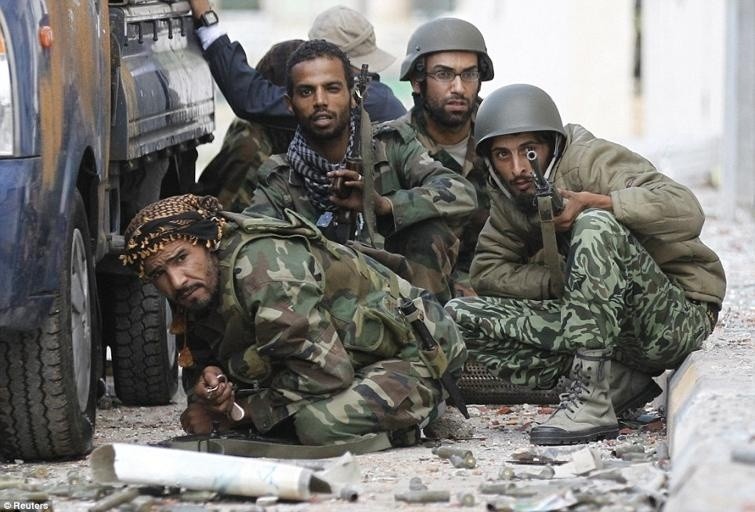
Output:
(0, 0), (219, 463)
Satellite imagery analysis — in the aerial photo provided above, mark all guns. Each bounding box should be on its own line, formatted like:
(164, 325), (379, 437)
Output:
(526, 150), (571, 257)
(316, 64), (368, 243)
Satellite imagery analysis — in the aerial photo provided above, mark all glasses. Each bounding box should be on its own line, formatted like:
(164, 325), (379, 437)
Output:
(423, 69), (484, 84)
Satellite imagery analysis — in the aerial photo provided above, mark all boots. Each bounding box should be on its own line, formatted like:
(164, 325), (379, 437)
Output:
(531, 345), (663, 445)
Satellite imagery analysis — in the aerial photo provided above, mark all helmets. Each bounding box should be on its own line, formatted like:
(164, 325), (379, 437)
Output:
(473, 83), (569, 157)
(399, 17), (496, 82)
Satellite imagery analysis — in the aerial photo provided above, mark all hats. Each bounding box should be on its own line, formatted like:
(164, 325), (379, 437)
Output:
(308, 5), (396, 72)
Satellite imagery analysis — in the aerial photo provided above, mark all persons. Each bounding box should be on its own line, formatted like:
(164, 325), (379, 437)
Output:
(122, 1), (727, 446)
